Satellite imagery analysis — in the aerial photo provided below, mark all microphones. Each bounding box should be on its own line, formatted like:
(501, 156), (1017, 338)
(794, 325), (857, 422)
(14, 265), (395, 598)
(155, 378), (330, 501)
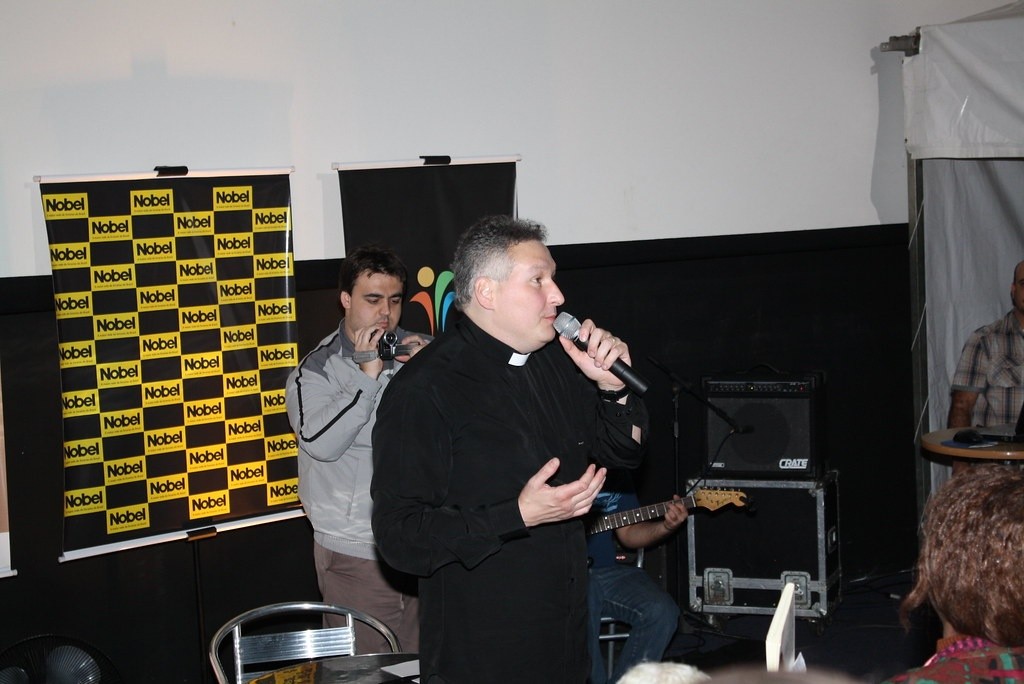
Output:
(552, 311), (651, 397)
(731, 425), (754, 432)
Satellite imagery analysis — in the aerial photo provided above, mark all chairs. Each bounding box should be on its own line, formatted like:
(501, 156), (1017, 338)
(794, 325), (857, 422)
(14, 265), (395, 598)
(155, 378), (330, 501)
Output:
(207, 604), (399, 684)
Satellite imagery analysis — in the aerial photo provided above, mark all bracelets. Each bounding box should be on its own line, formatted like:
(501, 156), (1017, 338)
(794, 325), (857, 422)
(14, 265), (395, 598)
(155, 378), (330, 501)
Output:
(663, 521), (676, 531)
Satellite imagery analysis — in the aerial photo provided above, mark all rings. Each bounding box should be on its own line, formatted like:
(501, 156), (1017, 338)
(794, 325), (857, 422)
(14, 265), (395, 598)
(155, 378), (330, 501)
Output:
(609, 336), (616, 342)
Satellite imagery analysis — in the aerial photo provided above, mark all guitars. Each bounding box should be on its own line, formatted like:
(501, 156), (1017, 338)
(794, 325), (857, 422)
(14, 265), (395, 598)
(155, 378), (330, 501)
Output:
(582, 486), (750, 538)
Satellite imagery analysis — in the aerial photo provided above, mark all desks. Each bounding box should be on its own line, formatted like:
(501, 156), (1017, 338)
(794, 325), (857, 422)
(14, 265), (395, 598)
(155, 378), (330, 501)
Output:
(687, 472), (842, 622)
(922, 426), (1024, 459)
(246, 652), (420, 684)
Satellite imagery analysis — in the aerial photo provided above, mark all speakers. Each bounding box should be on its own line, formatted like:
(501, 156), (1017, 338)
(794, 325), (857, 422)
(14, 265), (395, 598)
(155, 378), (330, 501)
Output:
(702, 371), (828, 479)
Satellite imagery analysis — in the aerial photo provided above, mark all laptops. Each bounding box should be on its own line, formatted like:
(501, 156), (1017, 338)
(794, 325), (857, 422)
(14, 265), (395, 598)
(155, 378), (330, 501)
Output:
(976, 401), (1024, 444)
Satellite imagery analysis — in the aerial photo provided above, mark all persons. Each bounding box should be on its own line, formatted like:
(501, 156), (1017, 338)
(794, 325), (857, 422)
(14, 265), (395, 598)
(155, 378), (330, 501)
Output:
(370, 215), (651, 684)
(883, 462), (1024, 684)
(587, 493), (688, 684)
(286, 241), (435, 655)
(946, 258), (1024, 475)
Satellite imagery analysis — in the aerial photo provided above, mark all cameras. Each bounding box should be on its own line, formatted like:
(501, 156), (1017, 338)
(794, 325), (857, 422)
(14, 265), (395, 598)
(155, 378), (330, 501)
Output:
(368, 329), (421, 360)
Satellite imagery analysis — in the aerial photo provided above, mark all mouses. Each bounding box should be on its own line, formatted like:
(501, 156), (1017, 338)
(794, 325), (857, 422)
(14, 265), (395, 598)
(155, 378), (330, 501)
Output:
(952, 428), (985, 444)
